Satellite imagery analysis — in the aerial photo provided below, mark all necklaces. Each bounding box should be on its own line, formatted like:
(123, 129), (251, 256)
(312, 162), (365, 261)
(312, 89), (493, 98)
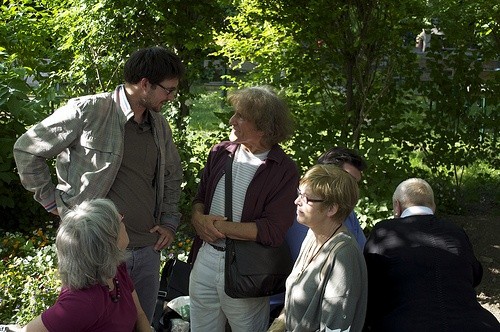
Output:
(308, 223), (343, 265)
(107, 276), (121, 303)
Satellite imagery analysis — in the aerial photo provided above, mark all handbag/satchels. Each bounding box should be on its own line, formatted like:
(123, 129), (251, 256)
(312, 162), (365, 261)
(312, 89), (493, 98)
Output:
(224, 235), (294, 299)
(158, 258), (193, 302)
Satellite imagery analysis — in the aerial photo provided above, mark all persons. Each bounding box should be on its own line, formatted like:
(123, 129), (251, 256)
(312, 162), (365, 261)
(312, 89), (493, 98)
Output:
(19, 198), (155, 332)
(184, 87), (500, 332)
(12, 46), (185, 326)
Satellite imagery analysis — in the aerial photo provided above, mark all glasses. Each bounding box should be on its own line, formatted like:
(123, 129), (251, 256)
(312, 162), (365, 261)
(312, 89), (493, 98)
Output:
(296, 188), (325, 205)
(148, 79), (175, 97)
